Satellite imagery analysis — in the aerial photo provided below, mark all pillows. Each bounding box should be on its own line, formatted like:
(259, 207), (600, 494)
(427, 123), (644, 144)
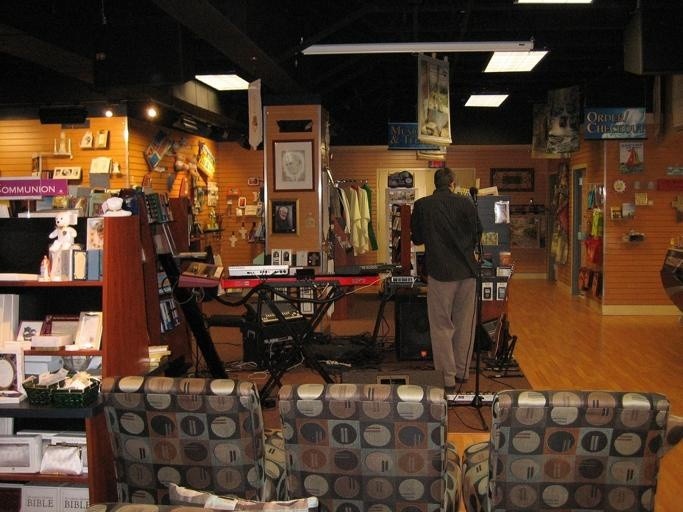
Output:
(163, 477), (310, 511)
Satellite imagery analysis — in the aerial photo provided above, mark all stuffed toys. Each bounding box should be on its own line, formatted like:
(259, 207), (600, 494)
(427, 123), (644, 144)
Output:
(100, 198), (132, 217)
(48, 212), (77, 245)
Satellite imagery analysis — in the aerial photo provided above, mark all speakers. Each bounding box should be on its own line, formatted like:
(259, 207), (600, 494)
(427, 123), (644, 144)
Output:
(396, 297), (432, 360)
(475, 317), (498, 352)
(623, 0), (683, 75)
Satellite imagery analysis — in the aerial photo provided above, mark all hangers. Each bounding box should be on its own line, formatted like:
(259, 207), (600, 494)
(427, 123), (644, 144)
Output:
(329, 178), (370, 192)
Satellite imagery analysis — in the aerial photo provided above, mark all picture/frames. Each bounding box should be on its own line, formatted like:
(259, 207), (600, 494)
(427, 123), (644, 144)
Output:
(52, 166), (82, 181)
(246, 176), (260, 186)
(237, 196), (247, 209)
(271, 139), (315, 193)
(13, 318), (45, 343)
(73, 310), (103, 351)
(94, 127), (110, 150)
(225, 185), (241, 197)
(488, 165), (536, 193)
(267, 197), (301, 237)
(43, 311), (80, 341)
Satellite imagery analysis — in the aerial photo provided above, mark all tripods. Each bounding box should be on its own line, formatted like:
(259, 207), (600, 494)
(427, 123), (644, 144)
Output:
(447, 207), (494, 430)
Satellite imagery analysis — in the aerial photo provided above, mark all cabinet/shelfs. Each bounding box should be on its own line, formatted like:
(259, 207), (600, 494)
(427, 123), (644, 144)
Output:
(0, 206), (152, 512)
(609, 214), (646, 245)
(140, 191), (199, 371)
(391, 202), (410, 278)
(384, 171), (417, 279)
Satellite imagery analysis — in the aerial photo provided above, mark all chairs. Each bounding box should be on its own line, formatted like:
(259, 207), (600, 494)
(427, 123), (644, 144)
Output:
(97, 371), (287, 506)
(274, 380), (459, 512)
(458, 387), (676, 511)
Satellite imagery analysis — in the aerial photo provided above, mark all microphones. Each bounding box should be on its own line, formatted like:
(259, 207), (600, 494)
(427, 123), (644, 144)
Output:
(470, 187), (478, 207)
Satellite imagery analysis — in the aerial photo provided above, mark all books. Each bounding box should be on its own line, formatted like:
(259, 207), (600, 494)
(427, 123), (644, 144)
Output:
(154, 234), (180, 330)
(148, 344), (172, 367)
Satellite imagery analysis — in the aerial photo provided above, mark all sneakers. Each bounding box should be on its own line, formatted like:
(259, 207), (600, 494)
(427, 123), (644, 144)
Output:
(455, 377), (468, 384)
(445, 386), (455, 393)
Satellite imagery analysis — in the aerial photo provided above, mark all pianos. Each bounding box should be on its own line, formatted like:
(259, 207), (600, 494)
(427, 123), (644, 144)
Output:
(220, 265), (390, 289)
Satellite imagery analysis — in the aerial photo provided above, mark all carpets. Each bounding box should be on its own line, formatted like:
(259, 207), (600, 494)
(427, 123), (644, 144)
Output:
(333, 331), (532, 392)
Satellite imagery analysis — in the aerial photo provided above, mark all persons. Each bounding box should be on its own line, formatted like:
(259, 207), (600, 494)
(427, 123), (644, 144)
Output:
(273, 206), (290, 231)
(408, 166), (483, 390)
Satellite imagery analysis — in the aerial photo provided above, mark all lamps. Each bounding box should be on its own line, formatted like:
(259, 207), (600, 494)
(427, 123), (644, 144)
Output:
(296, 38), (541, 61)
